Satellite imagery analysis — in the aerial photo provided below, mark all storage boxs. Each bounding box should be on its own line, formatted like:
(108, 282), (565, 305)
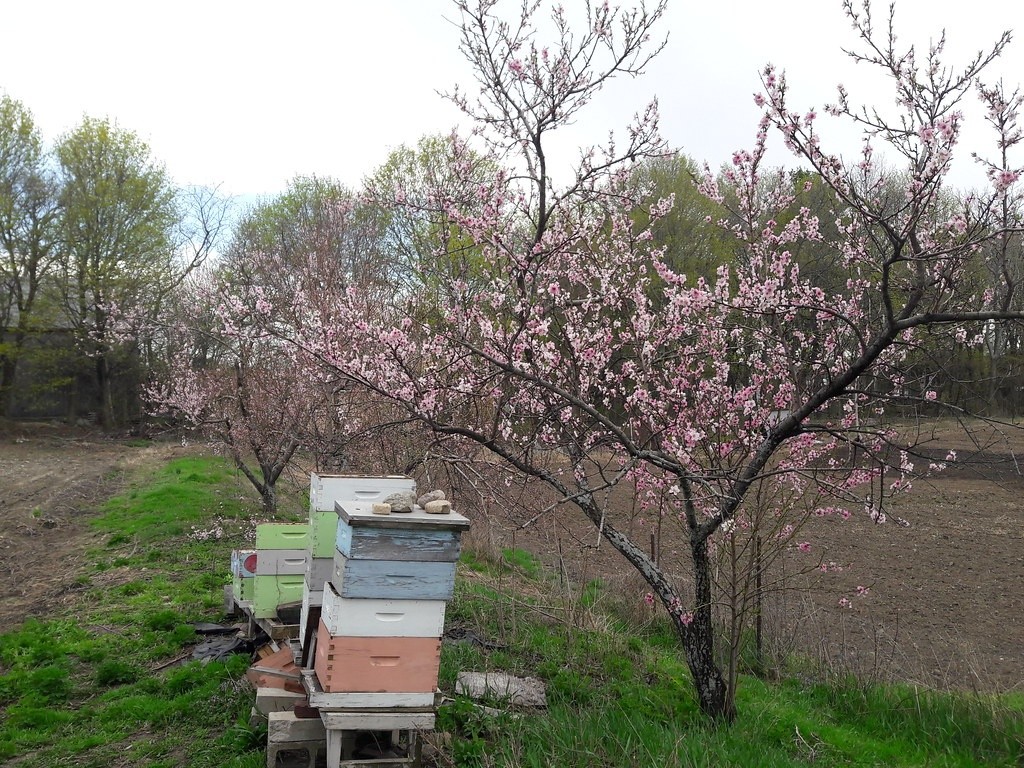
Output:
(230, 471), (469, 708)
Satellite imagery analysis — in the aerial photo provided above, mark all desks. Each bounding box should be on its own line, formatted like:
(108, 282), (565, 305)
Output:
(233, 597), (437, 768)
